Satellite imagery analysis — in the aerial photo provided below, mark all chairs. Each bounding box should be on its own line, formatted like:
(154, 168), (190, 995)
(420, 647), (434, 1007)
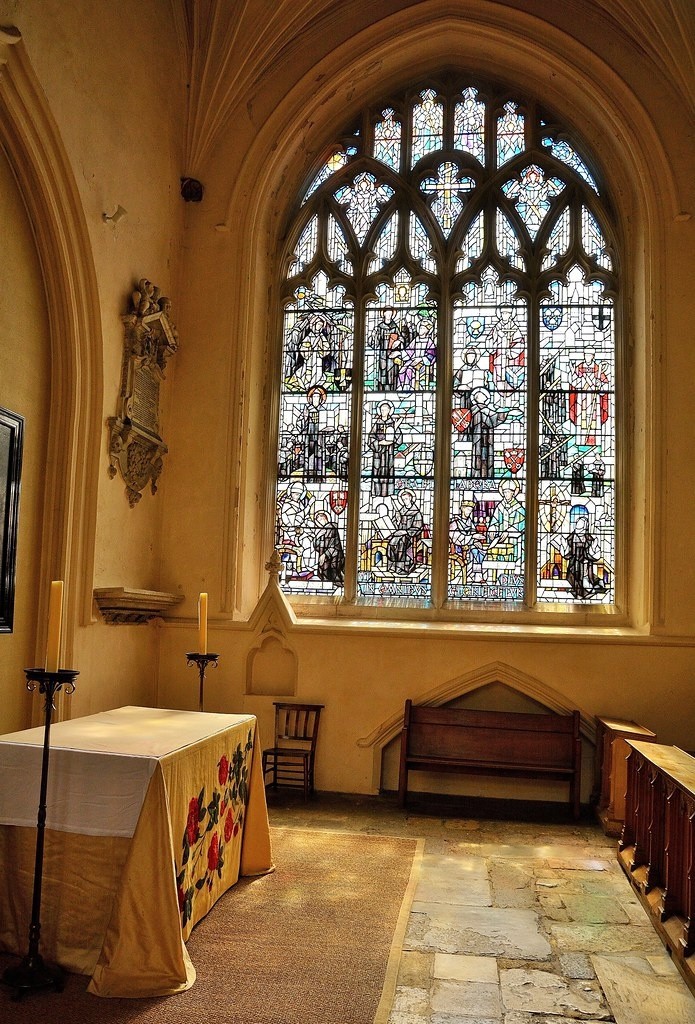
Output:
(263, 702), (325, 808)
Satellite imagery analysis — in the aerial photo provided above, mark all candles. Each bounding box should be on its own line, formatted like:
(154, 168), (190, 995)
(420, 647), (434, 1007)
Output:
(196, 592), (208, 656)
(43, 581), (63, 673)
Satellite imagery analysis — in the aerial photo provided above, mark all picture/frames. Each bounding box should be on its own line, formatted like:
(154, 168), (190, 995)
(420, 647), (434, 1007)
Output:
(0, 405), (26, 636)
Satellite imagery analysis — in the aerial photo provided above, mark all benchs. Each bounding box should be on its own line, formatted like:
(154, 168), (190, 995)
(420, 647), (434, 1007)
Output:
(399, 697), (582, 817)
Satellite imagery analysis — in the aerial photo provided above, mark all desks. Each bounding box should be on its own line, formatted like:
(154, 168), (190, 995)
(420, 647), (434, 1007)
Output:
(0, 704), (276, 998)
